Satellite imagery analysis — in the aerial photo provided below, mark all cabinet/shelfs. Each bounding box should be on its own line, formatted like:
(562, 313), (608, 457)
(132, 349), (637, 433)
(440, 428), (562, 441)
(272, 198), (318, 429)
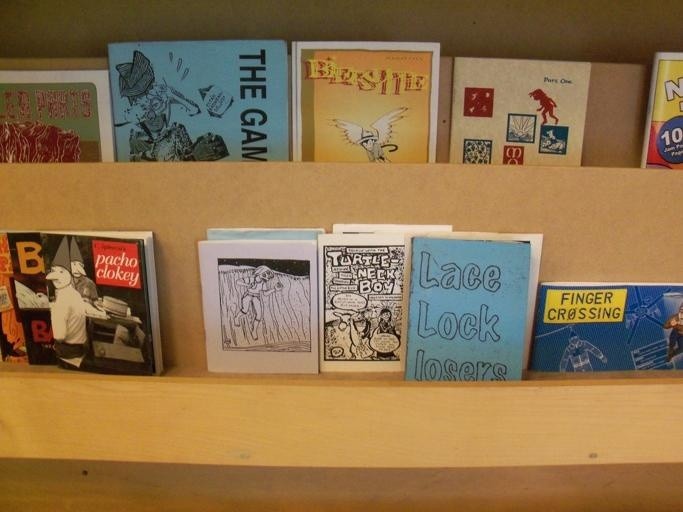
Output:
(0, 0), (683, 511)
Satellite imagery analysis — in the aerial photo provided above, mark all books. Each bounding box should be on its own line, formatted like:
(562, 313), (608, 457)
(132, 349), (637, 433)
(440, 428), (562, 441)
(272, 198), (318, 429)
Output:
(106, 39), (290, 163)
(448, 56), (592, 167)
(0, 230), (163, 376)
(292, 41), (440, 162)
(0, 70), (116, 163)
(640, 52), (683, 169)
(195, 224), (544, 382)
(528, 282), (683, 371)
